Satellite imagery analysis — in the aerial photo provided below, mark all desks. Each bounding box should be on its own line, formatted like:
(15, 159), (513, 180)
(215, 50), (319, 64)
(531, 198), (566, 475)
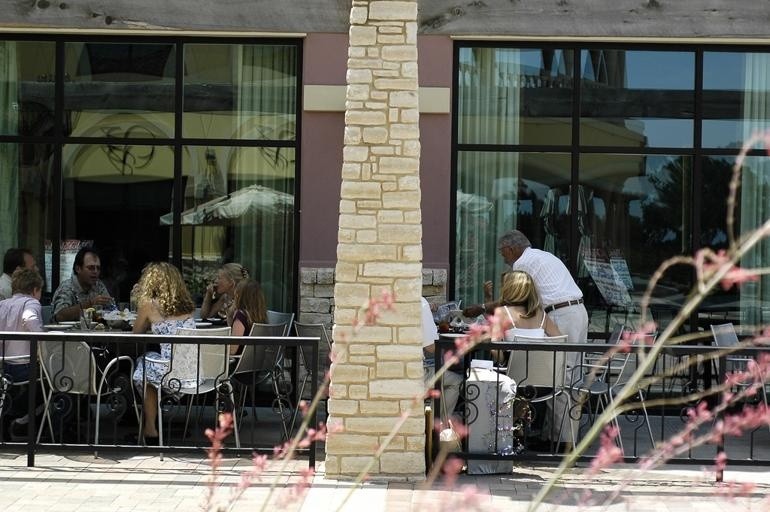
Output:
(3, 315), (233, 383)
(430, 320), (493, 403)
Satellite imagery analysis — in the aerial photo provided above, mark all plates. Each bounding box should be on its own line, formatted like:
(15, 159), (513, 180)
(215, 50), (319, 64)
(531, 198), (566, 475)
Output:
(59, 321), (98, 327)
(44, 324), (74, 331)
(195, 322), (212, 327)
(463, 330), (472, 335)
(194, 318), (222, 322)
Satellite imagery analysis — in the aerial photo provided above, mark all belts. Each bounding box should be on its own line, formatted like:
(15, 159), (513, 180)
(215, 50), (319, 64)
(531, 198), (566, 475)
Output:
(545, 298), (584, 313)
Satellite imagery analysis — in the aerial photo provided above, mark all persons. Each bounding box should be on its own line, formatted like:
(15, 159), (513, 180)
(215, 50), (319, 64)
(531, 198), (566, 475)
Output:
(201, 262), (269, 418)
(50, 249), (119, 419)
(1, 270), (75, 435)
(133, 260), (204, 445)
(421, 229), (589, 461)
(0, 245), (39, 299)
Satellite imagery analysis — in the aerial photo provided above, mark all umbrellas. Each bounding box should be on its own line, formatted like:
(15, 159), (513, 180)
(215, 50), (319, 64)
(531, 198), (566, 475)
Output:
(159, 184), (294, 227)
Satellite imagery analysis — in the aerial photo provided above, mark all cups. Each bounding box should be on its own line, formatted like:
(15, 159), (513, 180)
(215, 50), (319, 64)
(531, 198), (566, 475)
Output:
(91, 304), (103, 322)
(79, 308), (93, 330)
(449, 309), (463, 319)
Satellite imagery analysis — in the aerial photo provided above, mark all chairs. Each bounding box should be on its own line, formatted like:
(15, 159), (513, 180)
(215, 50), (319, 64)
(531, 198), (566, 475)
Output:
(424, 405), (435, 462)
(37, 331), (141, 446)
(139, 327), (241, 460)
(229, 321), (291, 455)
(288, 319), (332, 440)
(630, 302), (768, 463)
(242, 310), (296, 441)
(0, 343), (49, 440)
(502, 335), (579, 455)
(555, 322), (628, 457)
